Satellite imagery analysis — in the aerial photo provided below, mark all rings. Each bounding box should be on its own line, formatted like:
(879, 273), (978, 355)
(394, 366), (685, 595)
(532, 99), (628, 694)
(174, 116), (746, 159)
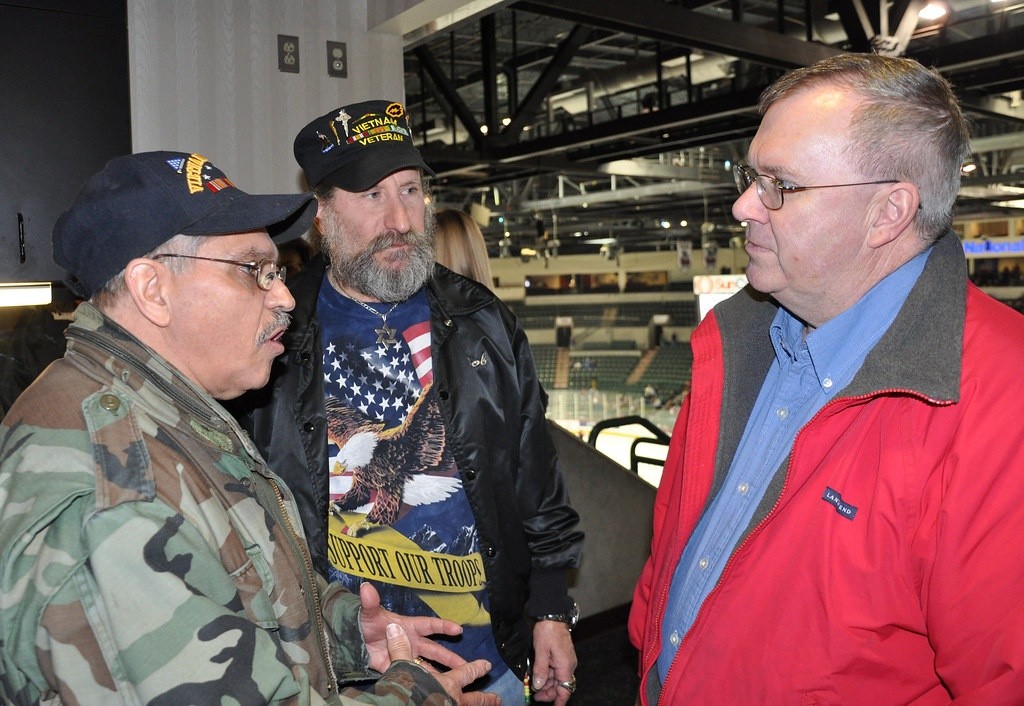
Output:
(559, 674), (577, 695)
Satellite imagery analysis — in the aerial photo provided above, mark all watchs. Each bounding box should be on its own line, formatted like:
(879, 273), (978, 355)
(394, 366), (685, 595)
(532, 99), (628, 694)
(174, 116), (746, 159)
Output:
(536, 600), (580, 632)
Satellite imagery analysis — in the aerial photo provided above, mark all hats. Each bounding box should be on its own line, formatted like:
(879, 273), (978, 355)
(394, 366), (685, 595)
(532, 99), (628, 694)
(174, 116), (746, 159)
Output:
(52, 150), (318, 302)
(294, 99), (438, 193)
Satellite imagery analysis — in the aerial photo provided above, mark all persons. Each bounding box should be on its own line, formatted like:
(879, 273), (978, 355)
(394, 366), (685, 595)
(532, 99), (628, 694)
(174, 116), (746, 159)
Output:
(627, 51), (1022, 706)
(0, 149), (503, 706)
(214, 100), (586, 706)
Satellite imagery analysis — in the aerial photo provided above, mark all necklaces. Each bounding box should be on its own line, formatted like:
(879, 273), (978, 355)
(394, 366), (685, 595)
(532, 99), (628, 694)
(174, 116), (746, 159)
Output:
(331, 267), (401, 350)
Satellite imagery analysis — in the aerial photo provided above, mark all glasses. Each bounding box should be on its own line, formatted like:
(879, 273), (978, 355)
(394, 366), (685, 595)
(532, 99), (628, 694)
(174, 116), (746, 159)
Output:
(152, 254), (287, 291)
(732, 163), (924, 211)
